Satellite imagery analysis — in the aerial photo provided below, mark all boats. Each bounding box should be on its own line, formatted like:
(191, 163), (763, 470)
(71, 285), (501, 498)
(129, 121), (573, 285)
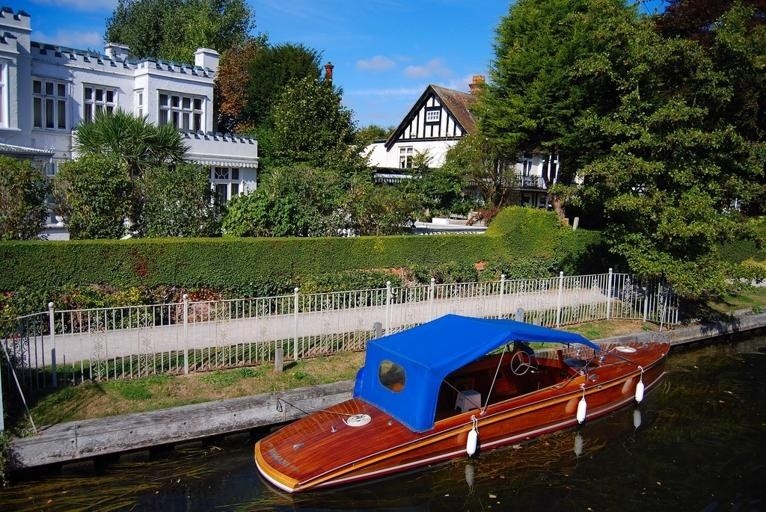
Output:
(253, 313), (671, 494)
